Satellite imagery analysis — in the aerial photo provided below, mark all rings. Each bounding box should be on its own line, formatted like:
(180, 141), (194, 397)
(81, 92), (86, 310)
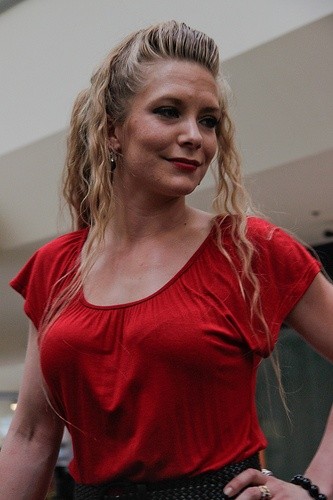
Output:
(259, 486), (273, 500)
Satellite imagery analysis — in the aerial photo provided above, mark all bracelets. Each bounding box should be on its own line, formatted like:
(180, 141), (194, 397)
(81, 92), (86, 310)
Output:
(290, 474), (328, 500)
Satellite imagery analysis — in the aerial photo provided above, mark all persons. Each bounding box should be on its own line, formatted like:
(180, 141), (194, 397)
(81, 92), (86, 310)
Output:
(1, 20), (333, 500)
